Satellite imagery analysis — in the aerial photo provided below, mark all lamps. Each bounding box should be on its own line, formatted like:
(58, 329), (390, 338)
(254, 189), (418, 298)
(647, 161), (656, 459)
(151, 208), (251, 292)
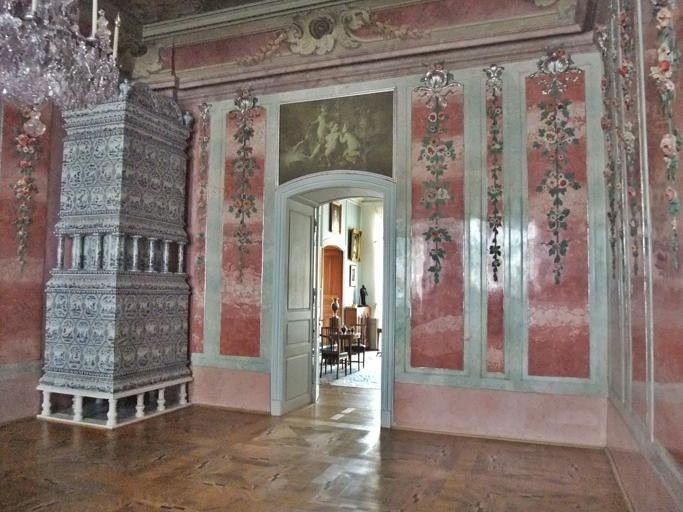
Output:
(0, 0), (123, 137)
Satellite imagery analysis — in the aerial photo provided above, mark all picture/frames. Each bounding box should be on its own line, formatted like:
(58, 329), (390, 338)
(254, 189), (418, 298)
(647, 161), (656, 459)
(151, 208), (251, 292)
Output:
(349, 228), (360, 261)
(329, 202), (341, 233)
(350, 265), (357, 288)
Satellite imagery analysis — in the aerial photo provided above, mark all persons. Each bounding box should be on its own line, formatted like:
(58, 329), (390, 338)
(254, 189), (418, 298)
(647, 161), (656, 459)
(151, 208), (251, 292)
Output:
(359, 284), (367, 305)
(299, 103), (370, 167)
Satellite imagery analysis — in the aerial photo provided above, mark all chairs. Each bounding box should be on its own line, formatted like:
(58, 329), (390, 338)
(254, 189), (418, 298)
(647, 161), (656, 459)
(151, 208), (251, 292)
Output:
(320, 326), (365, 379)
